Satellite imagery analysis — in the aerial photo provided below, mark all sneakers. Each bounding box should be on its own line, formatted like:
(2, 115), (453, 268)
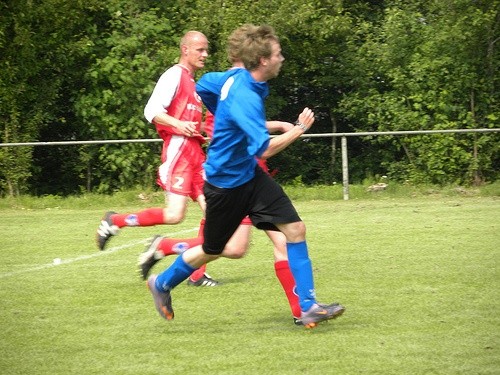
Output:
(146, 274), (174, 320)
(186, 274), (222, 287)
(135, 234), (170, 281)
(301, 304), (346, 329)
(95, 211), (119, 252)
(294, 302), (340, 325)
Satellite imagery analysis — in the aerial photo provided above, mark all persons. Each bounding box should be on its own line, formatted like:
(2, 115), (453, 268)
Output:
(94, 30), (222, 286)
(135, 27), (340, 324)
(145, 25), (344, 330)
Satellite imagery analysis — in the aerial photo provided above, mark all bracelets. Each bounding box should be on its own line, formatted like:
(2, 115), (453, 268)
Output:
(297, 121), (308, 132)
